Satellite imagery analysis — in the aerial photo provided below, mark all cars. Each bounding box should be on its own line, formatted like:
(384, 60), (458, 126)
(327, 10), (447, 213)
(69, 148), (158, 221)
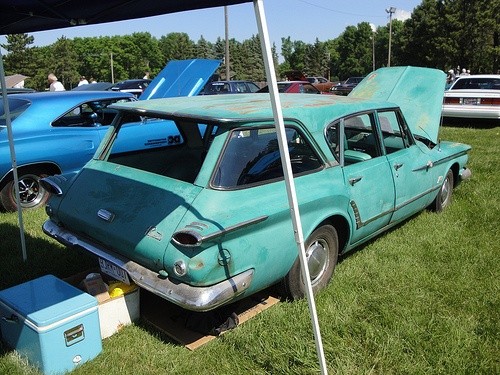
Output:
(36, 64), (473, 318)
(0, 57), (224, 216)
(0, 72), (366, 110)
(441, 73), (500, 127)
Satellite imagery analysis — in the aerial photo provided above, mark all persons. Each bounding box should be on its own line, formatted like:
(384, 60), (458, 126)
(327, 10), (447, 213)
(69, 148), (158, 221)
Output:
(92, 79), (97, 83)
(447, 68), (471, 83)
(48, 73), (65, 92)
(78, 76), (89, 86)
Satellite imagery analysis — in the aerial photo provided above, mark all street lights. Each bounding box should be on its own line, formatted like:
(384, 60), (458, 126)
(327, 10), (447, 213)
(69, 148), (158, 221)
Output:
(384, 6), (397, 66)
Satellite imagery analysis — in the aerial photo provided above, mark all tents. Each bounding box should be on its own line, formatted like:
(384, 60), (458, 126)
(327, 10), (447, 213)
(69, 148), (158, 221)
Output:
(0, 0), (328, 375)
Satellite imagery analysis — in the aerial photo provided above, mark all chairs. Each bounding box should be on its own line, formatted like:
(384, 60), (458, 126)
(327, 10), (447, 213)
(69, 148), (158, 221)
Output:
(345, 150), (371, 165)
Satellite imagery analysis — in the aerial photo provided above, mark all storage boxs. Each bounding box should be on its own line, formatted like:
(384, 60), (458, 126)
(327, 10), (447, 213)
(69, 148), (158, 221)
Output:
(66, 267), (140, 340)
(0, 275), (103, 375)
(81, 276), (111, 303)
